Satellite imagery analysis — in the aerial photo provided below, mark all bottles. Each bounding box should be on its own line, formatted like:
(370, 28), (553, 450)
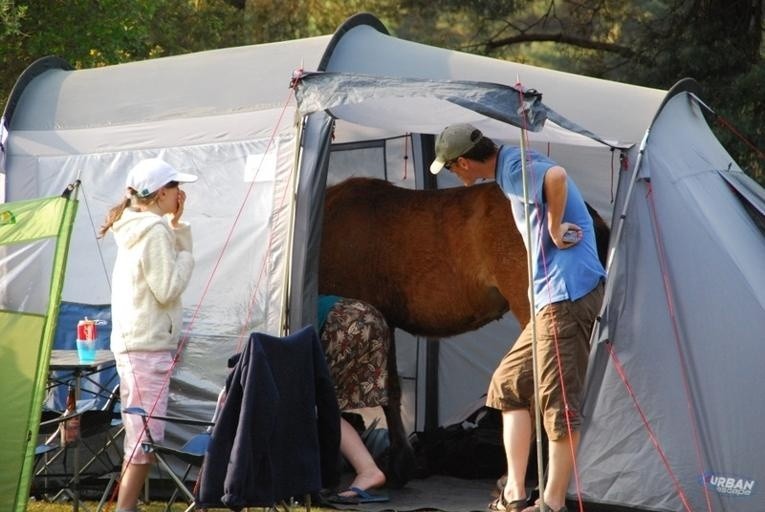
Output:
(59, 386), (80, 448)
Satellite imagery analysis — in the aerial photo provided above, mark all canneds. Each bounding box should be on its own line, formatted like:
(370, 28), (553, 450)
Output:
(77, 316), (97, 341)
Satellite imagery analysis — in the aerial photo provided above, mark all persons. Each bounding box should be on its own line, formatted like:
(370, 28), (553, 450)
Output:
(430, 124), (606, 511)
(316, 295), (391, 505)
(97, 157), (199, 511)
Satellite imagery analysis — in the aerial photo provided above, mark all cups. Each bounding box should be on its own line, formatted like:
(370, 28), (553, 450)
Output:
(77, 340), (97, 360)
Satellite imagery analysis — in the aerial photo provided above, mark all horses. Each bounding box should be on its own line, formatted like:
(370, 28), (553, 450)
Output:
(318, 177), (613, 493)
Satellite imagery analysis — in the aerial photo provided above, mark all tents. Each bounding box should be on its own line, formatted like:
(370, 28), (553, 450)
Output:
(0, 12), (764, 511)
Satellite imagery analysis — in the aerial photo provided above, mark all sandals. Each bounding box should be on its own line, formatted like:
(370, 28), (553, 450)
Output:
(488, 484), (569, 512)
(327, 486), (392, 503)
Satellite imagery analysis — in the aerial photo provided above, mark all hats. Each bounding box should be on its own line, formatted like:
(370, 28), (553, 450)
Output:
(124, 157), (200, 198)
(429, 122), (484, 176)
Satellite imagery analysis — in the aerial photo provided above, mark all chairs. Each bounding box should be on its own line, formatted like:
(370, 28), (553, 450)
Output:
(33, 297), (320, 511)
